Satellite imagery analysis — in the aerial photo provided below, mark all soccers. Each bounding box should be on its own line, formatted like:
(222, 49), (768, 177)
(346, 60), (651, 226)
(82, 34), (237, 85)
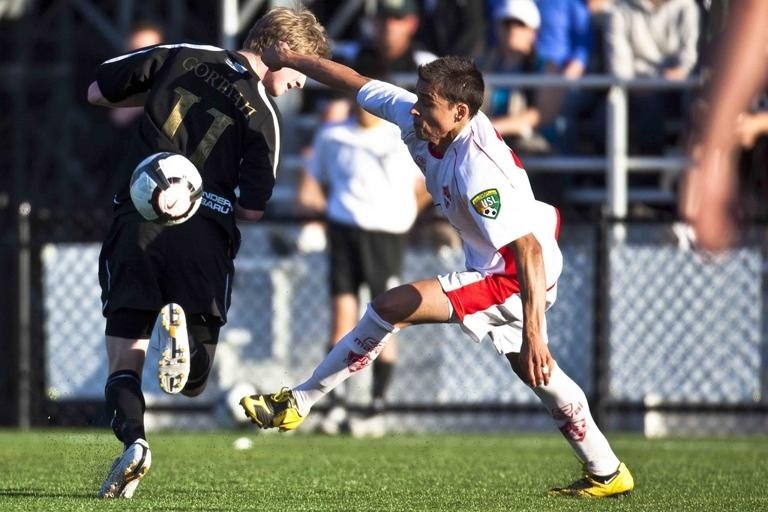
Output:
(130, 152), (204, 225)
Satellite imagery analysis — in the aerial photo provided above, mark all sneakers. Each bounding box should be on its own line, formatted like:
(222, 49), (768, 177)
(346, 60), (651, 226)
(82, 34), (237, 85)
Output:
(315, 404), (350, 437)
(97, 438), (152, 499)
(546, 461), (635, 500)
(150, 302), (191, 395)
(239, 387), (306, 433)
(344, 413), (384, 438)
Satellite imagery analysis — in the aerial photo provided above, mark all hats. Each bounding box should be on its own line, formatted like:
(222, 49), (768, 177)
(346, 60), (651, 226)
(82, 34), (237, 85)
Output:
(373, 0), (417, 20)
(497, 0), (540, 29)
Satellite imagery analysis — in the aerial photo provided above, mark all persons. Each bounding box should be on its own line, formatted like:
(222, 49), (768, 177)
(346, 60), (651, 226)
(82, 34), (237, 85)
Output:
(302, 88), (431, 437)
(242, 38), (637, 499)
(84, 5), (331, 496)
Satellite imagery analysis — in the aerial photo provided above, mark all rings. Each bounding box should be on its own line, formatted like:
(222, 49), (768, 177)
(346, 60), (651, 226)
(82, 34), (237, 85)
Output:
(541, 364), (550, 376)
